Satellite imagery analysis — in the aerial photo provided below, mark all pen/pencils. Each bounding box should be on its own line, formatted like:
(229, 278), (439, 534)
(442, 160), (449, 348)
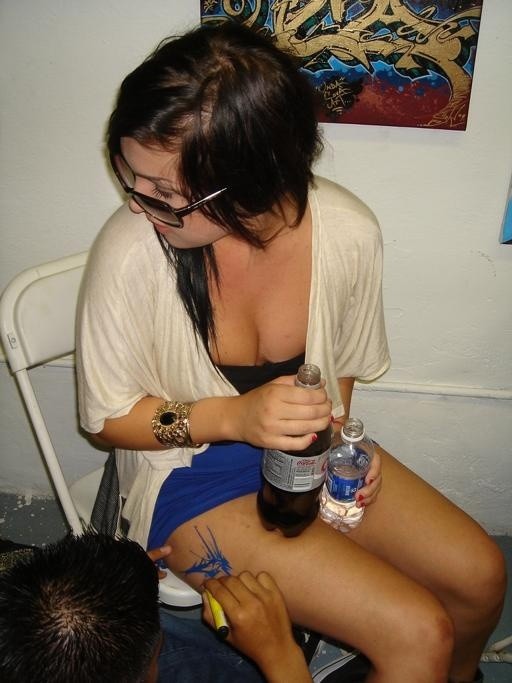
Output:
(204, 588), (231, 640)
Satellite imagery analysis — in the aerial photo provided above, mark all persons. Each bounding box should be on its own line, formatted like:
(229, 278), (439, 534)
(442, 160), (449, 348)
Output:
(74, 15), (509, 682)
(1, 534), (318, 682)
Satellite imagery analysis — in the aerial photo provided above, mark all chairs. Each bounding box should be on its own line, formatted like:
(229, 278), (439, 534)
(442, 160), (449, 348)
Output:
(1, 251), (203, 611)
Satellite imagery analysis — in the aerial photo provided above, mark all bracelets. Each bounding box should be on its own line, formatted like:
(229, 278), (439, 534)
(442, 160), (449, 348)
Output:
(151, 399), (205, 448)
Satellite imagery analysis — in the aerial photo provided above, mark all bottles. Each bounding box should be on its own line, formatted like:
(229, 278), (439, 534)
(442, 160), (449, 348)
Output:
(316, 417), (375, 533)
(255, 362), (332, 538)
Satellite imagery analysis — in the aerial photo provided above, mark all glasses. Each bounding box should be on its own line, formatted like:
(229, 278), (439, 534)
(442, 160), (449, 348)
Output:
(107, 152), (228, 229)
